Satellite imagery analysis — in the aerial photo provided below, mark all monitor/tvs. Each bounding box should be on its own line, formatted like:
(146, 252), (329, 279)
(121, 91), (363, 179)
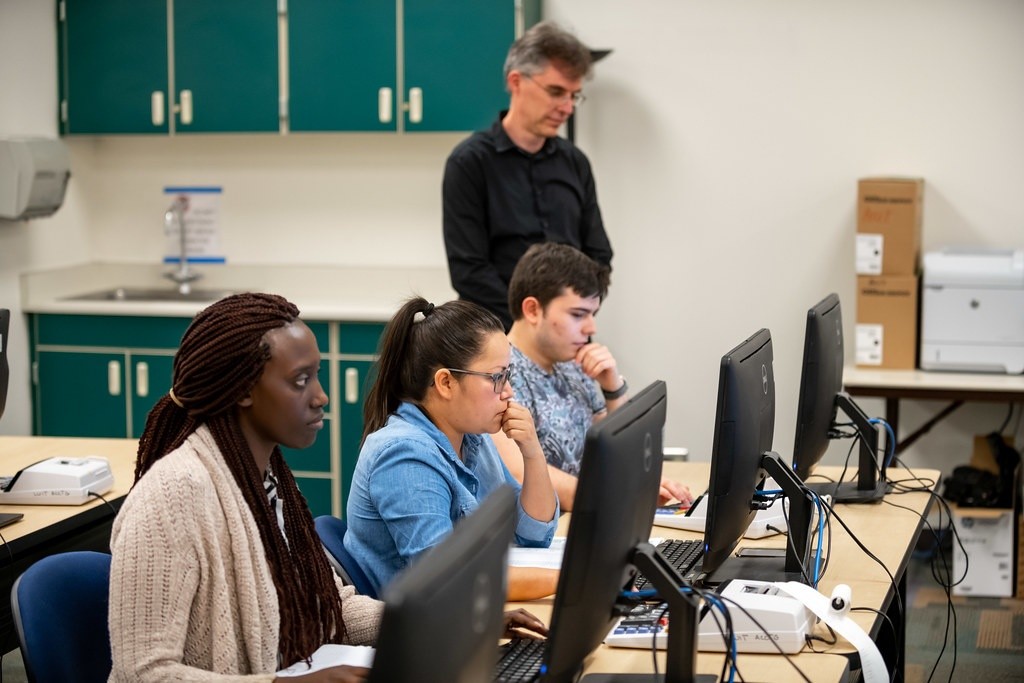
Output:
(0, 308), (9, 416)
(368, 293), (882, 683)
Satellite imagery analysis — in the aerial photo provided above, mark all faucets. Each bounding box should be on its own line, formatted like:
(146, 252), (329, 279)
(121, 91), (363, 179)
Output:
(163, 202), (194, 283)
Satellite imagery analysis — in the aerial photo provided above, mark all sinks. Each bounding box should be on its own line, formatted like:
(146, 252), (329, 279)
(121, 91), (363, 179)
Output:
(57, 286), (239, 303)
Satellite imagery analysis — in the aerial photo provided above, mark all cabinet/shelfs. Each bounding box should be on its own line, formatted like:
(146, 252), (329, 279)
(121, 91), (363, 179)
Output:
(26, 314), (389, 523)
(52, 0), (283, 138)
(283, 0), (544, 135)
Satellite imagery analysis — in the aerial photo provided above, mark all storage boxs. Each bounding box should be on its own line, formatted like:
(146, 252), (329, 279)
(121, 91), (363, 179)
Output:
(857, 179), (925, 368)
(930, 434), (1024, 598)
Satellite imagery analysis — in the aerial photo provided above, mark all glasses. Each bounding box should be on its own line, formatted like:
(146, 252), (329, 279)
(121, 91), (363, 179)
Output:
(522, 73), (586, 108)
(431, 363), (515, 394)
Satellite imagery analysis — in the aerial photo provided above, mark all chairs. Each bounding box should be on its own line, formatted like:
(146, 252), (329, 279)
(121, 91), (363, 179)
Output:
(310, 515), (376, 598)
(10, 550), (115, 683)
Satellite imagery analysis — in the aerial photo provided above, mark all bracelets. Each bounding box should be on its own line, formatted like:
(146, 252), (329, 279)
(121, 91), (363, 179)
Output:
(601, 375), (627, 401)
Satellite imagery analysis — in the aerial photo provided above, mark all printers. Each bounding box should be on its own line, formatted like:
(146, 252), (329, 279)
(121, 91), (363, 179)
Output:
(921, 247), (1023, 375)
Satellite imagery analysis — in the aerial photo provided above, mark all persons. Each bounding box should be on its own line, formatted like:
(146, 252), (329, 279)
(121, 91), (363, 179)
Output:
(435, 20), (613, 337)
(341, 296), (559, 601)
(485, 242), (694, 514)
(106, 294), (550, 682)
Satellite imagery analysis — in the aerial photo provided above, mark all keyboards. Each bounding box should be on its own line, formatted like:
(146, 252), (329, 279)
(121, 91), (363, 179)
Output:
(634, 539), (703, 590)
(492, 637), (549, 683)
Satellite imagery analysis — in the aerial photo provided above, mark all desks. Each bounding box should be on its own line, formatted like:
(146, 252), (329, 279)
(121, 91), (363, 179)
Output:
(842, 367), (1024, 467)
(0, 435), (147, 683)
(509, 464), (943, 683)
